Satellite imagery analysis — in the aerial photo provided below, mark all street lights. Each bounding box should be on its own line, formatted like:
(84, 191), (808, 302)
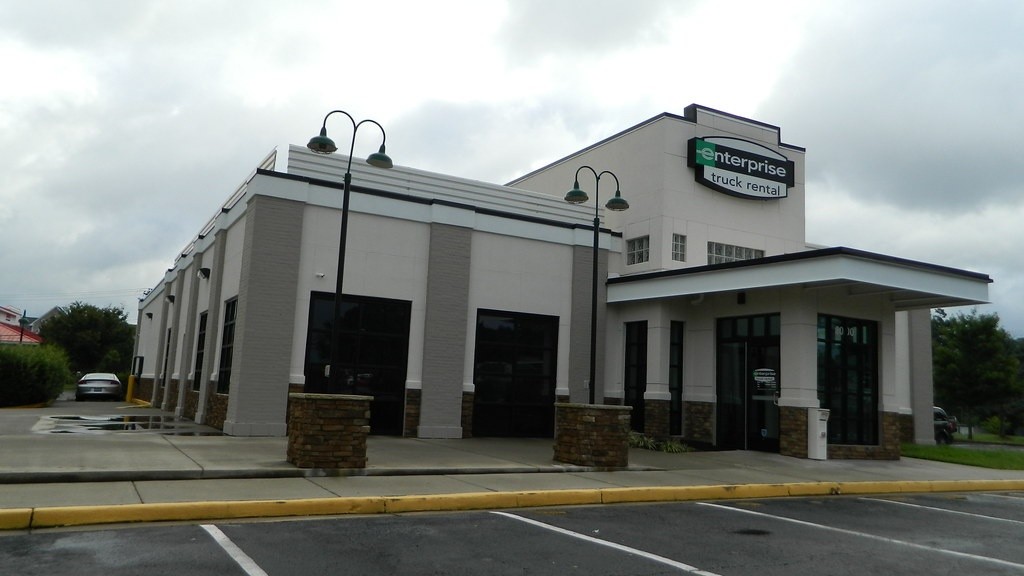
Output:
(563, 164), (631, 406)
(306, 109), (394, 395)
(18, 309), (29, 344)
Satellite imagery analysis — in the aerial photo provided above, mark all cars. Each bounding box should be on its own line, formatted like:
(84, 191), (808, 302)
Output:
(75, 372), (124, 401)
(934, 404), (958, 444)
